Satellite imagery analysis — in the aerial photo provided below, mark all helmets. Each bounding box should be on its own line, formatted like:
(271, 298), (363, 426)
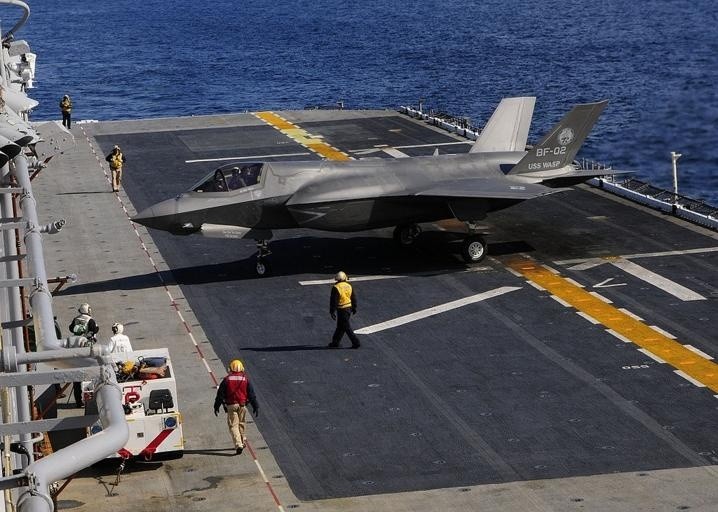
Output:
(112, 144), (120, 151)
(79, 302), (90, 315)
(112, 323), (124, 334)
(335, 270), (347, 281)
(230, 359), (243, 373)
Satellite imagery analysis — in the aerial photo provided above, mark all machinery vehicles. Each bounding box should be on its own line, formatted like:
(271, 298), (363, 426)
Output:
(76, 347), (189, 465)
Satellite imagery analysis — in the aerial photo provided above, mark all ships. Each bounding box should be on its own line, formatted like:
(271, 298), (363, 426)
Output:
(0, 0), (717, 512)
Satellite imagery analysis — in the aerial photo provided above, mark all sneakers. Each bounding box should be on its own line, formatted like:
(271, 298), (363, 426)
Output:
(236, 444), (246, 454)
(328, 341), (361, 349)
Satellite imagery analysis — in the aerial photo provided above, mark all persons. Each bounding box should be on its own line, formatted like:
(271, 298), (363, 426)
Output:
(226, 167), (247, 191)
(214, 359), (259, 454)
(59, 95), (71, 129)
(243, 165), (257, 186)
(328, 270), (360, 348)
(68, 302), (99, 344)
(107, 322), (133, 353)
(53, 316), (65, 398)
(64, 324), (90, 406)
(104, 145), (126, 192)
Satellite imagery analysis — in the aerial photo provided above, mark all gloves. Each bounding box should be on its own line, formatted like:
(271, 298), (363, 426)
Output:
(252, 406), (258, 417)
(331, 312), (337, 321)
(213, 406), (220, 417)
(351, 307), (357, 315)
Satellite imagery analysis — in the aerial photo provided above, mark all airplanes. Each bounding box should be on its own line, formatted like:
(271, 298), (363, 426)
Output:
(130, 96), (639, 279)
